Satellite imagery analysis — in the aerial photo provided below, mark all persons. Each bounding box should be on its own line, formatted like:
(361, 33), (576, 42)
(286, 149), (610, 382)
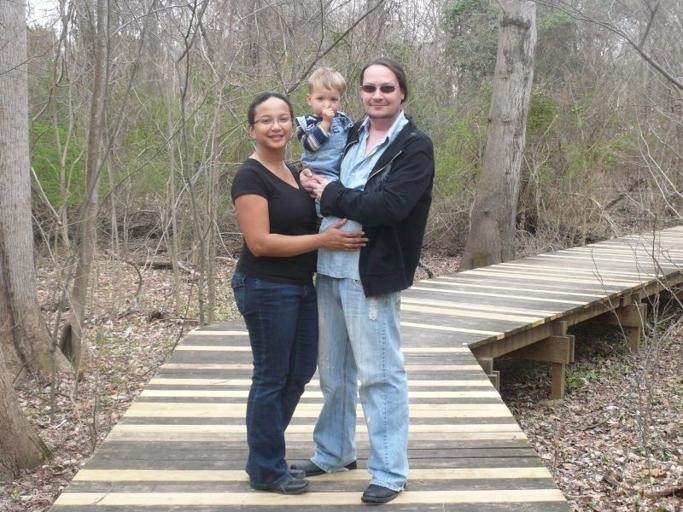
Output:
(228, 93), (369, 495)
(293, 65), (355, 233)
(289, 57), (435, 504)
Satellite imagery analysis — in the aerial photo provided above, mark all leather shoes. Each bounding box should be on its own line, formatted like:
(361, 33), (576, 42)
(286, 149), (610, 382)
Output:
(362, 484), (406, 503)
(291, 461), (356, 477)
(288, 468), (305, 478)
(271, 474), (309, 493)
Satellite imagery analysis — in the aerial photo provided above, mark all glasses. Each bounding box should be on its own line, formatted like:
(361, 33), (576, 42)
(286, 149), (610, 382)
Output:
(360, 85), (398, 93)
(253, 117), (291, 124)
